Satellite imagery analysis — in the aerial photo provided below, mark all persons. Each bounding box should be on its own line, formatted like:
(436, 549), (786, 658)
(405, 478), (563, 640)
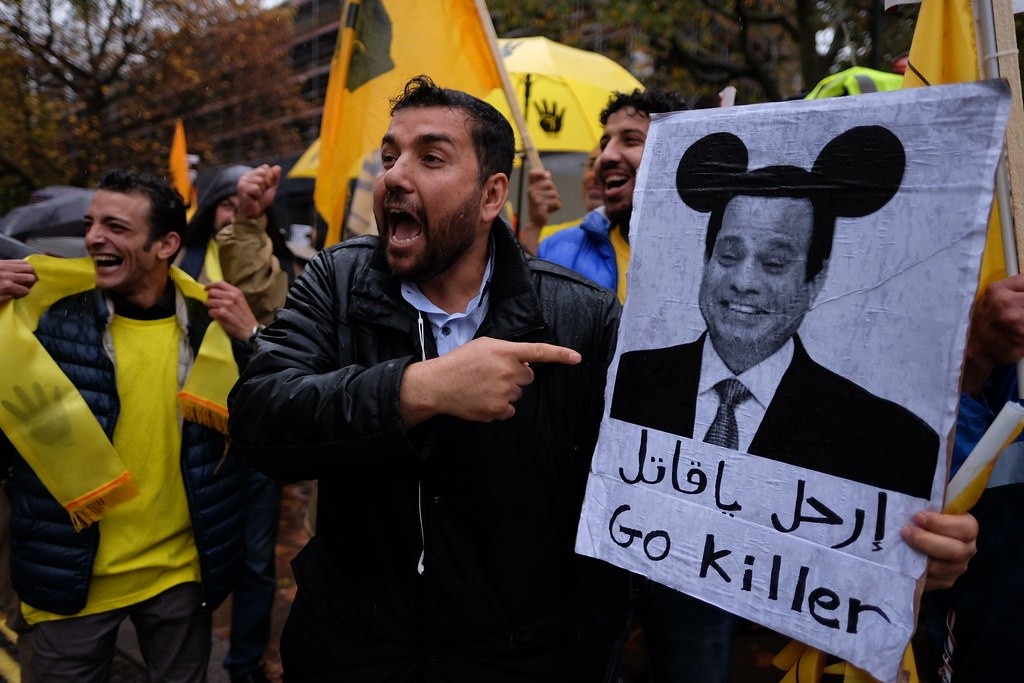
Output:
(516, 88), (688, 307)
(915, 268), (1024, 682)
(610, 166), (938, 499)
(173, 164), (288, 682)
(228, 75), (978, 682)
(1, 168), (267, 683)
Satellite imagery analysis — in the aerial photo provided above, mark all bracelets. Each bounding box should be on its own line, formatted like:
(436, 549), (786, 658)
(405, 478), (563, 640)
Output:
(249, 323), (266, 344)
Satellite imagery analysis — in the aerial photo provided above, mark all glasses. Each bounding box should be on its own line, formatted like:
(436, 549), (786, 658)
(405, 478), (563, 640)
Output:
(581, 155), (596, 173)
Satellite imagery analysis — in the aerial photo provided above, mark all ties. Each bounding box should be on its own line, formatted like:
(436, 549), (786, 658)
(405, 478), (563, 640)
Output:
(698, 378), (754, 453)
